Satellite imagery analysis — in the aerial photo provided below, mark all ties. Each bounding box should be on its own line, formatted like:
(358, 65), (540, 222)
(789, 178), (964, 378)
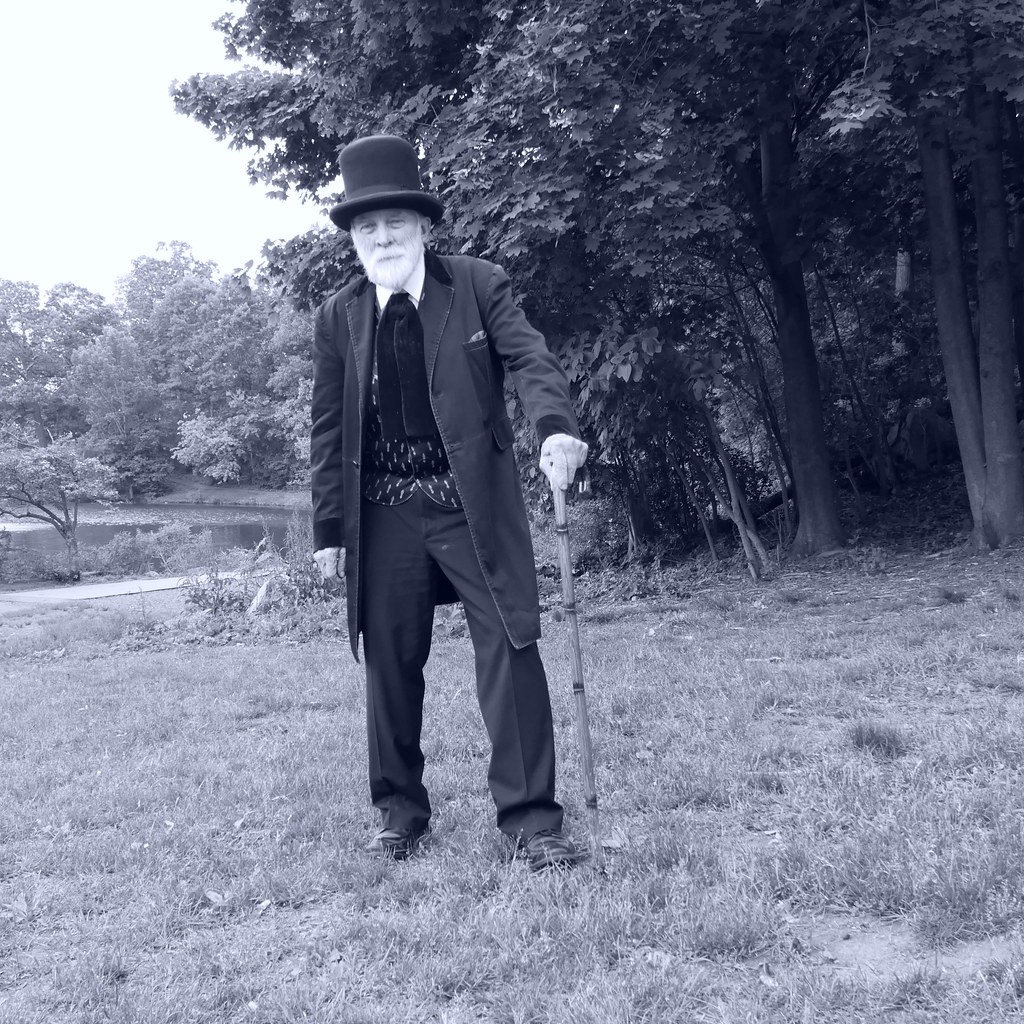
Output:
(376, 293), (436, 443)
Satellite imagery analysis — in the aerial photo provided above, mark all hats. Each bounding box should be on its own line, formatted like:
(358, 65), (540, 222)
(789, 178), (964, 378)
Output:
(330, 136), (445, 224)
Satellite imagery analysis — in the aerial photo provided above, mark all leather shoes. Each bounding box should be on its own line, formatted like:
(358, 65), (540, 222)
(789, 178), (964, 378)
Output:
(363, 823), (429, 862)
(521, 828), (576, 870)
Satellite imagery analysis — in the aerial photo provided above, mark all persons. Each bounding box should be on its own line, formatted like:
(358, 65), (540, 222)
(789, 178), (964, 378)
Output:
(311, 132), (588, 868)
